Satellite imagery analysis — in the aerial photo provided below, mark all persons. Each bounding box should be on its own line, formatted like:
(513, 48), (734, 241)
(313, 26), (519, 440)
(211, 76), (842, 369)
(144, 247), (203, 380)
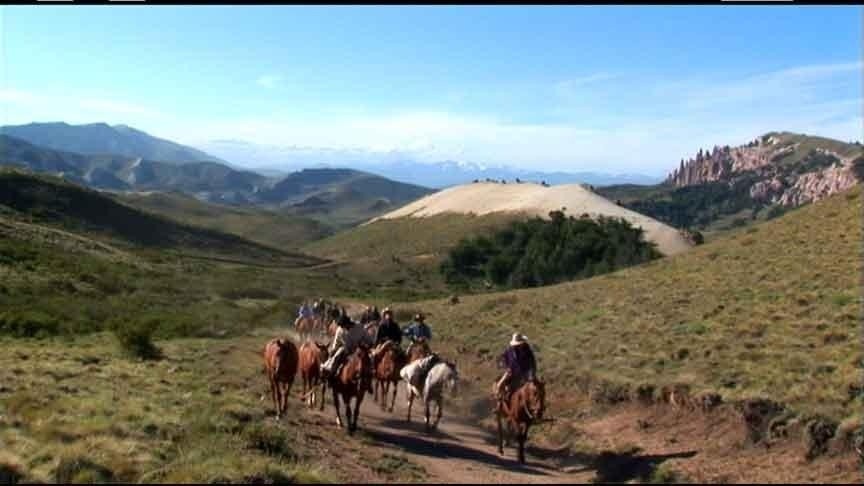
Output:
(295, 296), (431, 382)
(492, 332), (537, 414)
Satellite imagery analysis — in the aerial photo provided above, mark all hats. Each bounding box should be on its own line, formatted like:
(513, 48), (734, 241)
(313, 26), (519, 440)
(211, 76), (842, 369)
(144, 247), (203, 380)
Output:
(509, 333), (528, 347)
(382, 307), (392, 318)
(413, 314), (424, 320)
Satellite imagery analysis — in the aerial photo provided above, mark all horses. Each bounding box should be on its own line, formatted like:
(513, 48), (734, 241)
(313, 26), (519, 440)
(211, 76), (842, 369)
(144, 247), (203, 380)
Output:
(294, 313), (458, 436)
(260, 335), (299, 423)
(492, 372), (547, 464)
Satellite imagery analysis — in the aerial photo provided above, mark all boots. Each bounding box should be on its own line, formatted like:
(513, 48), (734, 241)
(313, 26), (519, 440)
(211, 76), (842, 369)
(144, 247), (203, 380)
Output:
(492, 401), (502, 412)
